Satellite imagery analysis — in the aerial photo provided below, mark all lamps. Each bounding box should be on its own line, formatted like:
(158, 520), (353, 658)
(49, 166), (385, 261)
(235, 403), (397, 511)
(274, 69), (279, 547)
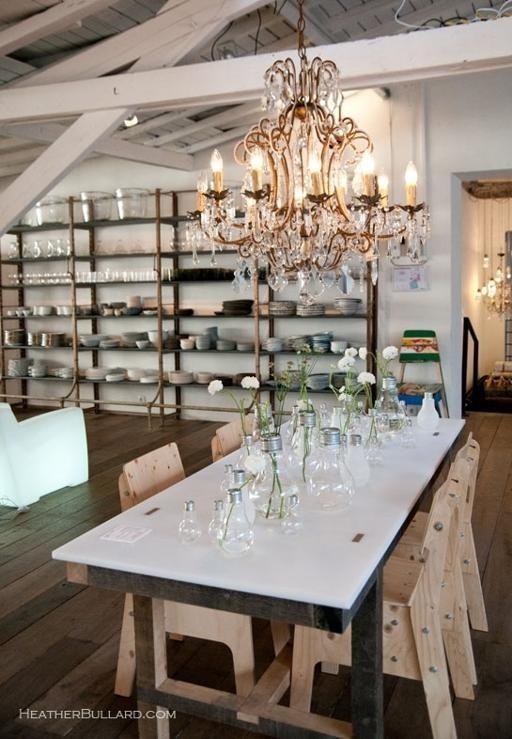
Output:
(185, 0), (431, 308)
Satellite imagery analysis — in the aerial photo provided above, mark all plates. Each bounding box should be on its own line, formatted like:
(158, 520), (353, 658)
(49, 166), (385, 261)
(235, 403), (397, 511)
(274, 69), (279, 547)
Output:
(262, 331), (349, 356)
(176, 308), (194, 316)
(8, 358), (75, 380)
(213, 299), (254, 316)
(3, 326), (69, 347)
(79, 329), (168, 349)
(216, 338), (254, 353)
(78, 363), (160, 385)
(335, 298), (362, 315)
(168, 369), (262, 388)
(266, 296), (324, 318)
(5, 303), (72, 317)
(282, 370), (356, 391)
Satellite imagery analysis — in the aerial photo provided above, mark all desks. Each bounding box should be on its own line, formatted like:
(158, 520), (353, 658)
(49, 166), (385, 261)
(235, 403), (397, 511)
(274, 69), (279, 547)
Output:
(51, 413), (466, 739)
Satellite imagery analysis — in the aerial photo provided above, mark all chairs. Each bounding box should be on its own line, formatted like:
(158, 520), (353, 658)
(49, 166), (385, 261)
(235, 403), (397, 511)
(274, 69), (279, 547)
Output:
(394, 329), (451, 420)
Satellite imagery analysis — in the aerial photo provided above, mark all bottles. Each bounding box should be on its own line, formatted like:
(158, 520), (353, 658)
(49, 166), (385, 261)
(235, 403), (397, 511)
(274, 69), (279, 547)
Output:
(206, 378), (412, 559)
(6, 237), (148, 259)
(178, 500), (201, 545)
(417, 392), (439, 431)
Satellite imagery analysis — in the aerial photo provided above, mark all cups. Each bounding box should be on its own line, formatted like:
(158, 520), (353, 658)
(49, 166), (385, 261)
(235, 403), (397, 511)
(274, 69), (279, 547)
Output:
(32, 186), (150, 225)
(6, 272), (72, 285)
(73, 267), (173, 281)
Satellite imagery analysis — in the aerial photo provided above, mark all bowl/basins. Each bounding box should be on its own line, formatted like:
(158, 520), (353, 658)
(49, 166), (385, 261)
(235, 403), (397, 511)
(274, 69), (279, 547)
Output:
(166, 326), (217, 351)
(79, 295), (160, 316)
(135, 341), (148, 349)
